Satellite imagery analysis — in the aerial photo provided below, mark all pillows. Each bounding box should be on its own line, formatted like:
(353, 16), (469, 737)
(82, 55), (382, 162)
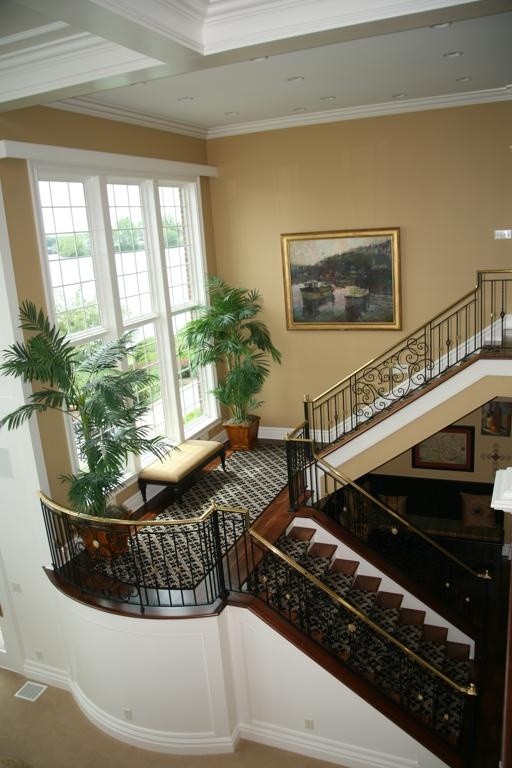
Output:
(459, 492), (495, 528)
(379, 493), (409, 520)
(343, 480), (372, 517)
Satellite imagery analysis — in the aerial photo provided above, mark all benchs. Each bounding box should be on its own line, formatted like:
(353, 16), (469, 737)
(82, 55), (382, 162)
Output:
(137, 439), (228, 509)
(323, 474), (505, 585)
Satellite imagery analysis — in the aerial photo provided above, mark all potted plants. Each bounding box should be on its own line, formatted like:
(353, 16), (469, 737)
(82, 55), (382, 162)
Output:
(0, 298), (183, 560)
(176, 273), (284, 450)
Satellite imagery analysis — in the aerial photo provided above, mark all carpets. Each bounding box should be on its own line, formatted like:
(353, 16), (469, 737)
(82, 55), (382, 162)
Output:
(93, 438), (315, 589)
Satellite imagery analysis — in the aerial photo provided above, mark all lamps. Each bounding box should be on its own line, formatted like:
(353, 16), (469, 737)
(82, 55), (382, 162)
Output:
(488, 466), (512, 561)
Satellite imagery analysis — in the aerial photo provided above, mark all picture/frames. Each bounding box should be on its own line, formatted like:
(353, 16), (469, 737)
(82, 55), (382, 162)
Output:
(410, 423), (476, 473)
(278, 226), (402, 334)
